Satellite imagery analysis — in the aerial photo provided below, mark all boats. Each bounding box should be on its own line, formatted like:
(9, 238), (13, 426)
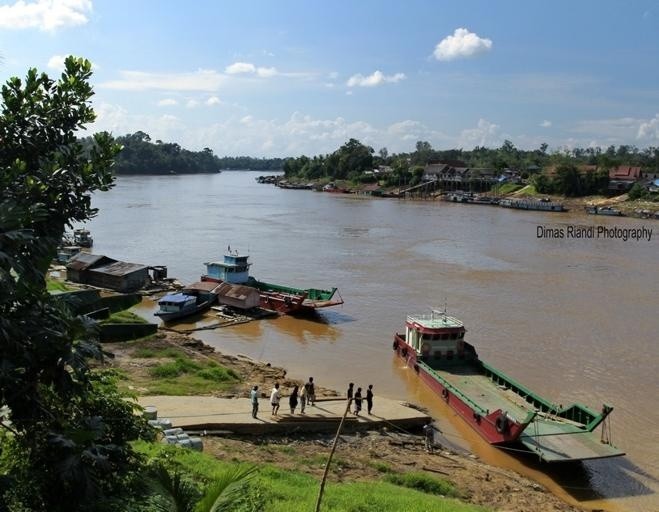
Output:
(586, 204), (621, 216)
(153, 282), (219, 323)
(199, 247), (344, 313)
(73, 227), (93, 248)
(390, 310), (625, 464)
(444, 190), (499, 204)
(500, 195), (569, 212)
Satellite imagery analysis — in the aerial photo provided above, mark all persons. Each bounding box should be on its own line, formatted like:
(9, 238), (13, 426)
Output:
(289, 385), (299, 414)
(299, 383), (310, 414)
(250, 385), (259, 418)
(353, 387), (363, 417)
(306, 376), (316, 406)
(270, 382), (282, 416)
(347, 383), (355, 414)
(366, 384), (373, 415)
